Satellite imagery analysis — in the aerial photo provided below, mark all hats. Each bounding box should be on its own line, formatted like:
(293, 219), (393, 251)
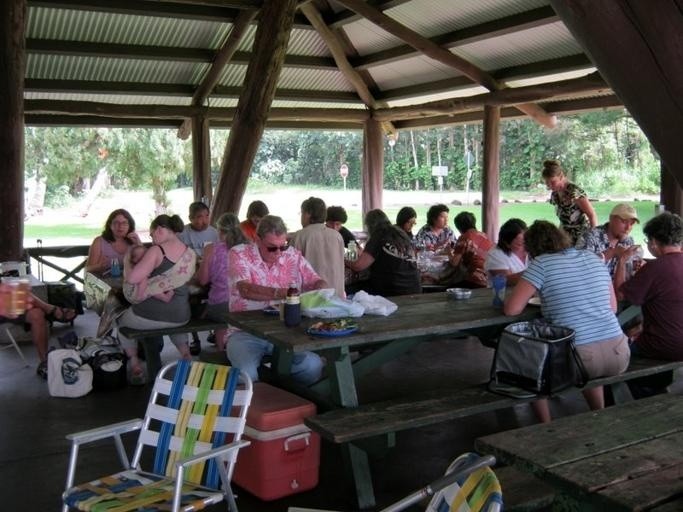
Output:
(610, 203), (639, 224)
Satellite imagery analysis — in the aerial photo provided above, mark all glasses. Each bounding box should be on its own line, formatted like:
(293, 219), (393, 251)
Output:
(644, 238), (650, 244)
(407, 220), (416, 225)
(148, 230), (156, 240)
(265, 239), (290, 252)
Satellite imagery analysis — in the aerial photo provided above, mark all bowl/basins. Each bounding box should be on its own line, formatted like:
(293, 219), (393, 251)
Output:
(446, 288), (472, 299)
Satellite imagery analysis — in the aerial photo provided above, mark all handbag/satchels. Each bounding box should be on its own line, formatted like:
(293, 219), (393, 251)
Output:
(47, 349), (93, 397)
(486, 320), (589, 400)
(440, 239), (472, 285)
(616, 300), (644, 338)
(300, 288), (365, 319)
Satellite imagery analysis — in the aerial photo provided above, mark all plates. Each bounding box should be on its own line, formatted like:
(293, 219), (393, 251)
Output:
(529, 297), (542, 305)
(264, 304), (280, 313)
(307, 320), (358, 337)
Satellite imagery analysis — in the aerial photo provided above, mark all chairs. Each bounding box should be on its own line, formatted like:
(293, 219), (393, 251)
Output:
(60, 358), (252, 511)
(0, 322), (31, 368)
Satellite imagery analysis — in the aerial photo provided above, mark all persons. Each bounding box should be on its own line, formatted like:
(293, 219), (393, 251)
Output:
(183, 197), (356, 387)
(0, 280), (32, 319)
(485, 202), (683, 423)
(396, 204), (492, 289)
(540, 157), (597, 251)
(0, 276), (77, 382)
(85, 209), (196, 386)
(342, 209), (419, 296)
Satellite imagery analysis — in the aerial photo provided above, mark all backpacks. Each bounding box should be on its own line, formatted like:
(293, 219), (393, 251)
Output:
(79, 335), (125, 374)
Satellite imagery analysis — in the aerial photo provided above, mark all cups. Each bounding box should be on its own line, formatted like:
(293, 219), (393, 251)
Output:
(279, 300), (287, 320)
(2, 277), (31, 315)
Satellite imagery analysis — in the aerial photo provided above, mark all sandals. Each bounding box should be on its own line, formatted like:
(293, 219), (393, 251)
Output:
(37, 361), (47, 379)
(190, 340), (200, 355)
(130, 365), (145, 386)
(207, 330), (215, 343)
(45, 305), (77, 322)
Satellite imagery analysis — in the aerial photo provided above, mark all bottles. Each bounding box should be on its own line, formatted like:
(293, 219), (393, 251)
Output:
(416, 240), (426, 262)
(491, 274), (506, 306)
(348, 241), (358, 261)
(285, 283), (301, 326)
(111, 256), (120, 276)
(630, 245), (644, 276)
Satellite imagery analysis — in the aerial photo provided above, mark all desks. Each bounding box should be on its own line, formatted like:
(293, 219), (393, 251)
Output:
(204, 286), (543, 511)
